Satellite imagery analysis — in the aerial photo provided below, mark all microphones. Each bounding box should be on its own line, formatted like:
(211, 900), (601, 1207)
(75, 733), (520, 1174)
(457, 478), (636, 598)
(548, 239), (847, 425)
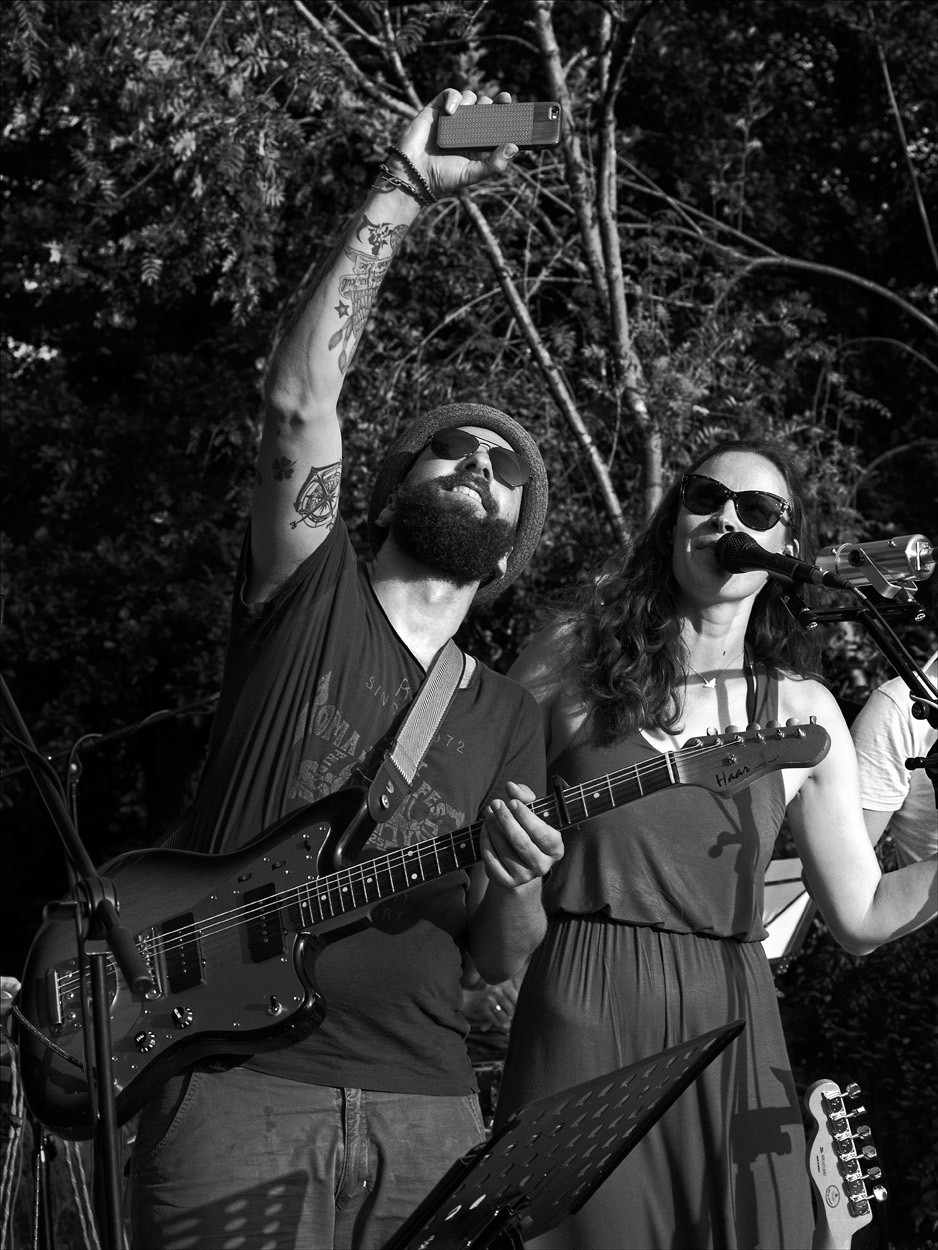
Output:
(815, 533), (936, 590)
(714, 531), (851, 593)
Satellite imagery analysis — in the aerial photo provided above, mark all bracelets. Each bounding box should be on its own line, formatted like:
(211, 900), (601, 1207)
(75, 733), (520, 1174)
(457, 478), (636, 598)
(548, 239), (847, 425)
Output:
(378, 144), (438, 207)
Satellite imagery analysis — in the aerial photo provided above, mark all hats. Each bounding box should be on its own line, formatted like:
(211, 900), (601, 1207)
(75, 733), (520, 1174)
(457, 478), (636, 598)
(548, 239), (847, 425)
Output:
(371, 403), (547, 606)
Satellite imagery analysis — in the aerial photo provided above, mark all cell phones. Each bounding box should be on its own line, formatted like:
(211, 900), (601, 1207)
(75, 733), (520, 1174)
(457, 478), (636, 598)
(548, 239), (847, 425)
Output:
(436, 101), (563, 153)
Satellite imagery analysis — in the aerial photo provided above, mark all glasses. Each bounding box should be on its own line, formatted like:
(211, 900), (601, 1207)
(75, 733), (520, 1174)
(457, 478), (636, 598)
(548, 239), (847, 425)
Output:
(424, 428), (534, 487)
(679, 475), (796, 532)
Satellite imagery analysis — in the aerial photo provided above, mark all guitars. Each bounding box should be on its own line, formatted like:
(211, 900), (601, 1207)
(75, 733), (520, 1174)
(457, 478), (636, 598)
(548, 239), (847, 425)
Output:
(16, 715), (832, 1141)
(804, 1078), (888, 1250)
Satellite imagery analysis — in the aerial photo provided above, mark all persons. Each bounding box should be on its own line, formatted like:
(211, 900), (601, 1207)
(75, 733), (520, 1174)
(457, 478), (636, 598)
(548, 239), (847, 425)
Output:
(851, 561), (938, 1250)
(125, 89), (564, 1249)
(508, 434), (938, 1250)
